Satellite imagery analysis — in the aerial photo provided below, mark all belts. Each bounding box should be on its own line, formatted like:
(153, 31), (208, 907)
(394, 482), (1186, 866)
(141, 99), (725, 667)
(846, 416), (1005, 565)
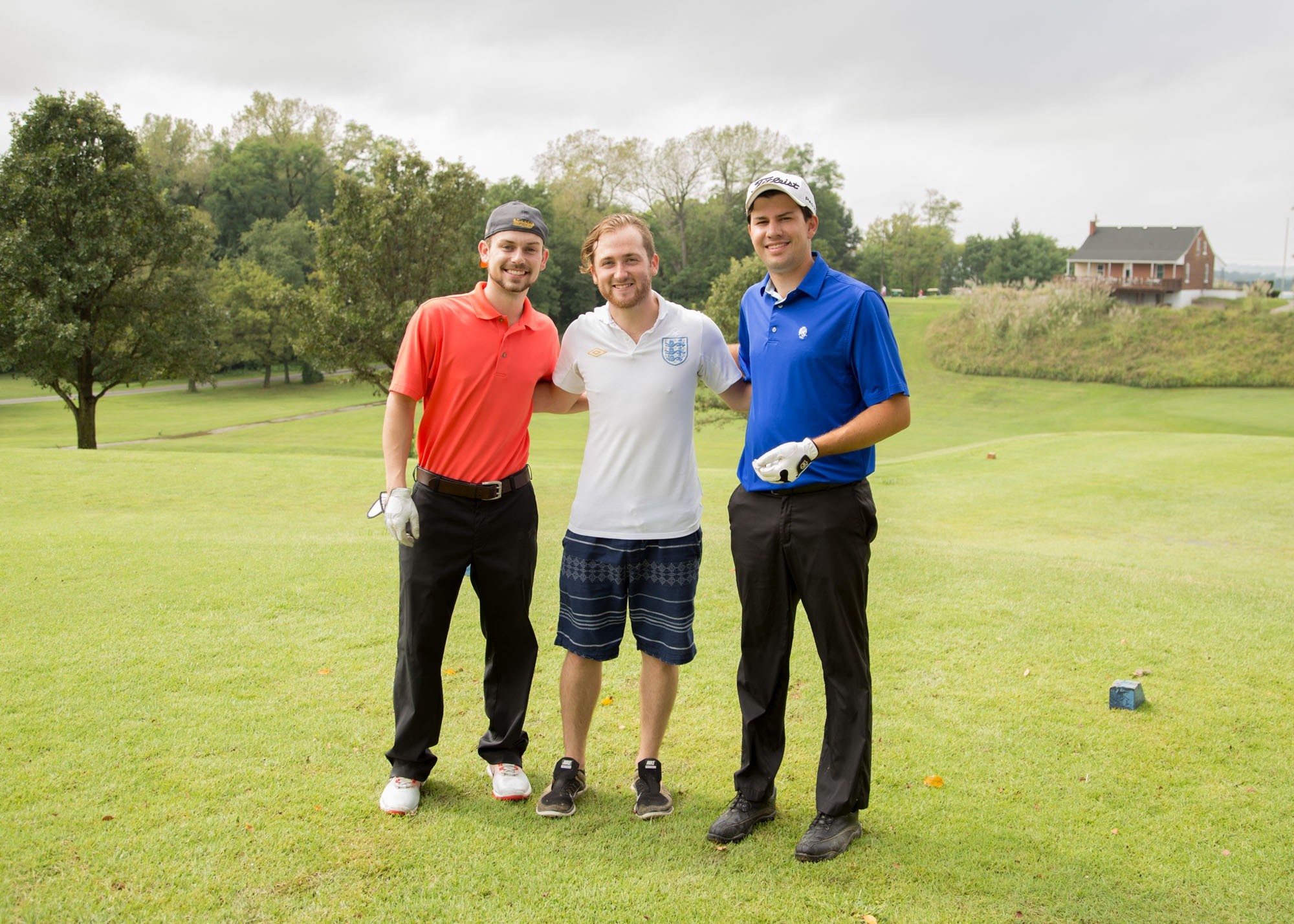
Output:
(416, 466), (531, 500)
(766, 482), (843, 495)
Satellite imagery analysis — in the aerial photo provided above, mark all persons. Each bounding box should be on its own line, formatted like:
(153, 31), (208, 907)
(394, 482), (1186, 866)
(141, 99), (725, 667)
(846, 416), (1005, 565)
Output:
(366, 169), (911, 863)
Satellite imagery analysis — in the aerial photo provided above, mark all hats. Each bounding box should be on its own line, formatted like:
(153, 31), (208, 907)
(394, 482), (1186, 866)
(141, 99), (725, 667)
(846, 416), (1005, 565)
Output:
(479, 201), (549, 269)
(745, 171), (816, 217)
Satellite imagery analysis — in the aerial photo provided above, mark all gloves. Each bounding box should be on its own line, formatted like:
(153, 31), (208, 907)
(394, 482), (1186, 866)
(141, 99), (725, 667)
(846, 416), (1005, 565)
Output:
(751, 437), (819, 485)
(367, 487), (419, 548)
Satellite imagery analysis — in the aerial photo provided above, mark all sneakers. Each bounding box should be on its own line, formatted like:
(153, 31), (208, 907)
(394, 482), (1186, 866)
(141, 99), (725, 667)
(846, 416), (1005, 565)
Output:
(537, 757), (586, 816)
(486, 763), (533, 799)
(379, 776), (426, 816)
(632, 758), (674, 818)
(706, 787), (777, 843)
(794, 810), (862, 862)
